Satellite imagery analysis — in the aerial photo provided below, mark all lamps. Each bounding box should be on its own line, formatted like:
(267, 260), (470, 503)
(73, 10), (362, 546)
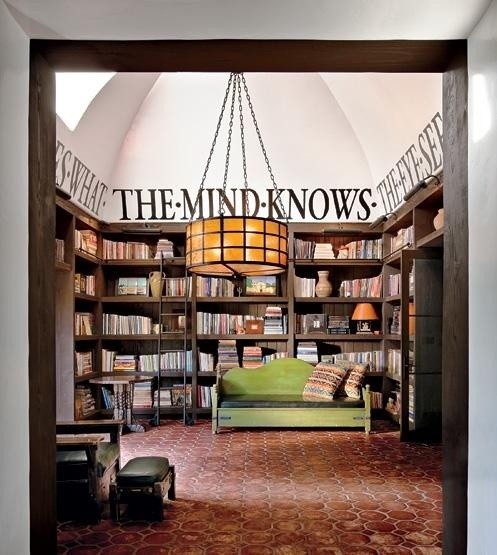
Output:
(185, 72), (289, 276)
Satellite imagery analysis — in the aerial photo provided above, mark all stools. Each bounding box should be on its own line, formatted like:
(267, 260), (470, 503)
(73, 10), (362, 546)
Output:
(109, 456), (176, 522)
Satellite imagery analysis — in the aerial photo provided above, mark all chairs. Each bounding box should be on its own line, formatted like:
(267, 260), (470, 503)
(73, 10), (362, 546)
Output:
(55, 420), (126, 524)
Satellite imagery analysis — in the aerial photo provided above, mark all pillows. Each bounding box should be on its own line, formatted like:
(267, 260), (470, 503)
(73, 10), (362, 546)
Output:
(303, 361), (351, 399)
(335, 360), (370, 399)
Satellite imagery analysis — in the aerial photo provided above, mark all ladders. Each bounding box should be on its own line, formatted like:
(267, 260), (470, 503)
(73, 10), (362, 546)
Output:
(156, 251), (187, 426)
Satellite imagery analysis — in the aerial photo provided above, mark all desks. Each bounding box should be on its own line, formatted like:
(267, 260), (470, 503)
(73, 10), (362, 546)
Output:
(88, 372), (154, 434)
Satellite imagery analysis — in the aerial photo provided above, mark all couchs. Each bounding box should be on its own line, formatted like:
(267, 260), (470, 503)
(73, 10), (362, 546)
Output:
(210, 358), (371, 435)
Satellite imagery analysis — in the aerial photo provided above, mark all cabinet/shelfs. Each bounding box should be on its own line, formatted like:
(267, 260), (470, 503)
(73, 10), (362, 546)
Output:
(292, 228), (385, 412)
(74, 220), (100, 420)
(382, 212), (415, 431)
(102, 228), (194, 414)
(193, 225), (291, 408)
(55, 208), (73, 267)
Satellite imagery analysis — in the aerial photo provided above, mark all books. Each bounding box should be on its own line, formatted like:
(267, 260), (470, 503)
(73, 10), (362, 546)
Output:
(56, 239), (64, 262)
(74, 273), (96, 296)
(117, 278), (146, 295)
(161, 350), (192, 406)
(197, 276), (289, 408)
(75, 230), (176, 260)
(101, 313), (158, 410)
(166, 276), (192, 297)
(75, 312), (95, 417)
(385, 225), (415, 423)
(293, 237), (383, 371)
(370, 391), (382, 409)
